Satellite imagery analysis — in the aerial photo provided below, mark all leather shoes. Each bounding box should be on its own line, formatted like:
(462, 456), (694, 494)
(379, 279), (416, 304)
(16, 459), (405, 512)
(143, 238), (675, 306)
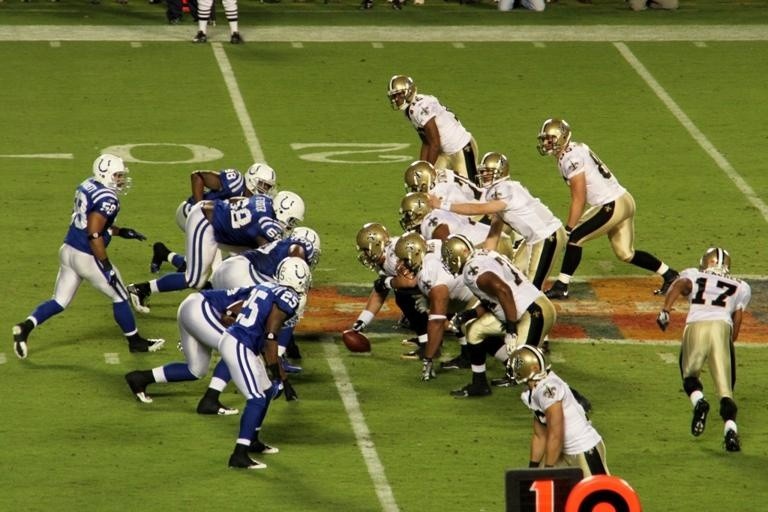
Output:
(658, 310), (670, 330)
(422, 358), (436, 380)
(411, 294), (431, 314)
(272, 375), (299, 404)
(119, 227), (147, 241)
(100, 259), (118, 283)
(351, 319), (367, 332)
(456, 309), (478, 326)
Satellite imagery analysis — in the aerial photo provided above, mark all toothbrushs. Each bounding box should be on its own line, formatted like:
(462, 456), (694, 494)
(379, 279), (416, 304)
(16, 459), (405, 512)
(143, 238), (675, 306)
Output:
(341, 330), (371, 354)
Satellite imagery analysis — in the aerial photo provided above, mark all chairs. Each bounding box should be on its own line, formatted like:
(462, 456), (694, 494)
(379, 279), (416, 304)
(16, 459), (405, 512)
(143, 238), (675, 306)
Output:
(126, 283), (150, 314)
(440, 355), (470, 369)
(125, 370), (152, 403)
(544, 286), (568, 300)
(228, 439), (278, 469)
(230, 33), (243, 44)
(725, 430), (740, 452)
(692, 399), (709, 436)
(150, 241), (164, 272)
(450, 384), (491, 398)
(196, 398), (239, 415)
(12, 324), (29, 358)
(654, 269), (679, 295)
(129, 337), (164, 353)
(400, 337), (443, 359)
(192, 30), (207, 42)
(492, 370), (517, 387)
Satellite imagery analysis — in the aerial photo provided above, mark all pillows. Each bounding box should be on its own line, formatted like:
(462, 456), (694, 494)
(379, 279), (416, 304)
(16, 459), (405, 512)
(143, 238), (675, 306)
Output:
(274, 190), (321, 295)
(537, 118), (572, 156)
(93, 154), (132, 195)
(475, 152), (509, 188)
(699, 248), (731, 276)
(395, 161), (437, 271)
(244, 163), (276, 195)
(356, 223), (389, 271)
(387, 74), (417, 111)
(507, 344), (552, 385)
(441, 234), (474, 279)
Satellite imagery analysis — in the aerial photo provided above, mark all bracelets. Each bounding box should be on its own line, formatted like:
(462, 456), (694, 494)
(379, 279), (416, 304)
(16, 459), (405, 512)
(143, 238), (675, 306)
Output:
(564, 226), (572, 236)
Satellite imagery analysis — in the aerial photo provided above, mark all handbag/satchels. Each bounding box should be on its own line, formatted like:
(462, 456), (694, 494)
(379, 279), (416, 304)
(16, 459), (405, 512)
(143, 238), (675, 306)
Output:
(564, 226), (572, 236)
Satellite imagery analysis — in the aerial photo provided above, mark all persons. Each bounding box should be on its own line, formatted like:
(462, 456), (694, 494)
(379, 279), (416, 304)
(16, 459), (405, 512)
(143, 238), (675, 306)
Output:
(505, 345), (609, 480)
(656, 247), (753, 450)
(156, 0), (679, 43)
(537, 119), (680, 298)
(128, 162), (320, 414)
(343, 150), (566, 397)
(217, 256), (313, 470)
(11, 154), (165, 358)
(385, 74), (482, 198)
